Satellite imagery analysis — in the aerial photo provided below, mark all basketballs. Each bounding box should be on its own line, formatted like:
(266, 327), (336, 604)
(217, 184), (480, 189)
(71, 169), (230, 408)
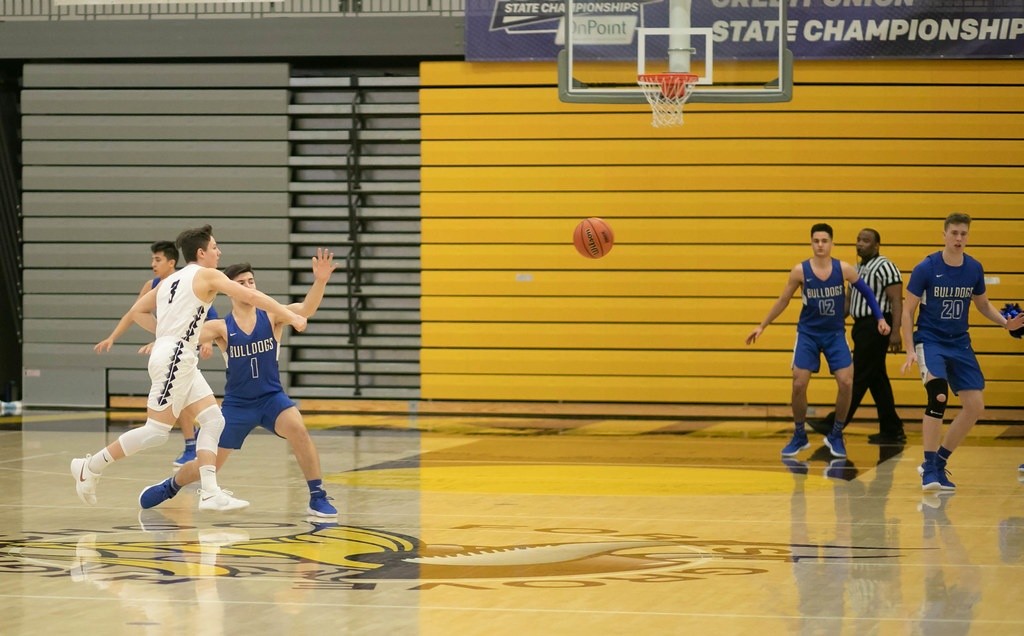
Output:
(571, 216), (616, 260)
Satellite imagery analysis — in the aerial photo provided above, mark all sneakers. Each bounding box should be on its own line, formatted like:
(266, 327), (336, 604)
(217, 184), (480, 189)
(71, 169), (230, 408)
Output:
(918, 461), (956, 491)
(197, 527), (249, 548)
(823, 431), (846, 456)
(917, 492), (954, 523)
(1018, 475), (1024, 484)
(806, 417), (839, 436)
(138, 508), (180, 533)
(868, 427), (904, 441)
(781, 429), (810, 456)
(781, 457), (808, 474)
(306, 518), (338, 534)
(173, 444), (197, 466)
(1018, 464), (1024, 471)
(71, 533), (103, 582)
(197, 489), (250, 511)
(139, 477), (175, 509)
(70, 454), (102, 507)
(307, 490), (338, 517)
(824, 458), (847, 479)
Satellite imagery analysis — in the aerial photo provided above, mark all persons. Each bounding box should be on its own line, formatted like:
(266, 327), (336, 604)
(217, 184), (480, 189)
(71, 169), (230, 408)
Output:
(70, 222), (308, 512)
(745, 221), (891, 456)
(91, 240), (220, 467)
(135, 246), (338, 520)
(71, 510), (339, 636)
(901, 212), (1024, 491)
(804, 227), (909, 444)
(781, 440), (984, 636)
